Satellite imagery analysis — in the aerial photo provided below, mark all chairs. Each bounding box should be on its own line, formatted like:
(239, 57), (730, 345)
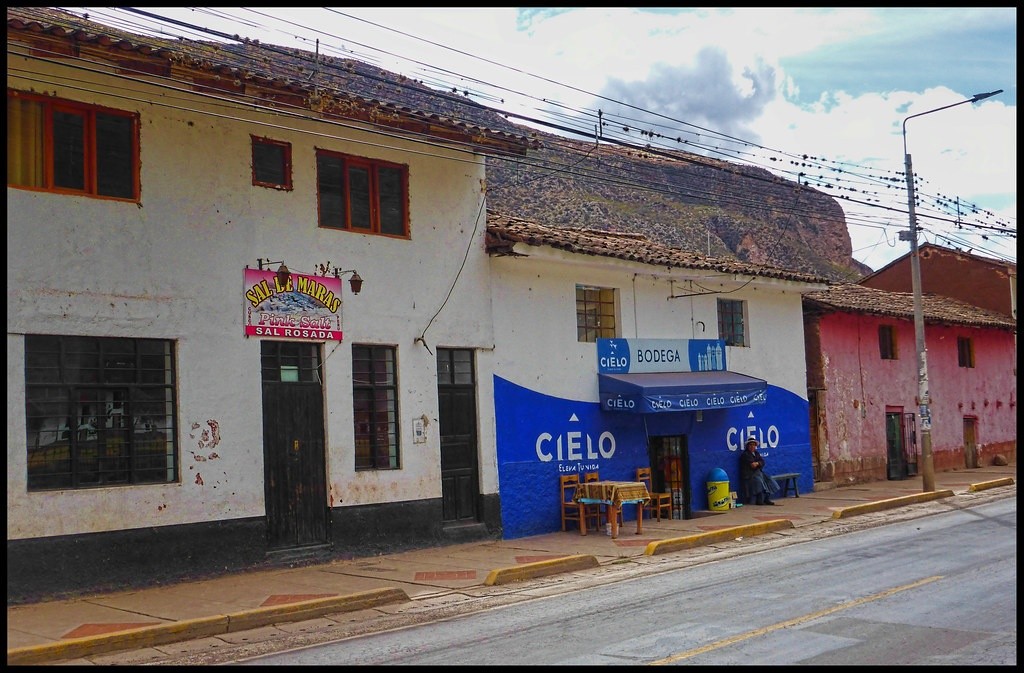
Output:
(584, 471), (623, 529)
(637, 466), (672, 522)
(560, 473), (600, 531)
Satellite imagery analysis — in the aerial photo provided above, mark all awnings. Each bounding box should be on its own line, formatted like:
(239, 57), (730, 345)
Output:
(597, 370), (769, 412)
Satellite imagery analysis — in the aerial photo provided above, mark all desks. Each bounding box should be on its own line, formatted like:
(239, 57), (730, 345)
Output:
(572, 480), (651, 540)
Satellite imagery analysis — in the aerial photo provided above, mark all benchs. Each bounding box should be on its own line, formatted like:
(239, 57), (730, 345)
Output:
(768, 473), (802, 498)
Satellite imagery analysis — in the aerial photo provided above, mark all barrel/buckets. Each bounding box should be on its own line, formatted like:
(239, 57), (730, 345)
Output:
(606, 523), (619, 535)
(707, 481), (730, 511)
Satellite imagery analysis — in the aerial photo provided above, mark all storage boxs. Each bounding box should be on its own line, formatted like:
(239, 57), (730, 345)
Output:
(664, 455), (682, 519)
(729, 492), (737, 508)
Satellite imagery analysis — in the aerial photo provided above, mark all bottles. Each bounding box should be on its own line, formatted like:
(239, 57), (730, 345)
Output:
(698, 353), (702, 371)
(712, 346), (716, 369)
(716, 343), (722, 370)
(702, 355), (704, 371)
(704, 354), (708, 371)
(707, 344), (712, 370)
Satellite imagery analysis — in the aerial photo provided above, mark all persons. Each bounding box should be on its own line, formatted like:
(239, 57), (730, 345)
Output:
(740, 437), (780, 506)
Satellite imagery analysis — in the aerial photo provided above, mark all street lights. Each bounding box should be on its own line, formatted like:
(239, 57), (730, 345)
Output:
(902, 89), (1005, 493)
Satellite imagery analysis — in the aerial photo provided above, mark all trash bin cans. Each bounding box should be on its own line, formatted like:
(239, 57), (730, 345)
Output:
(707, 467), (730, 511)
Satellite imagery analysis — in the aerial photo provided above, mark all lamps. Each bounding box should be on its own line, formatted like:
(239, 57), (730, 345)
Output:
(334, 267), (364, 296)
(257, 256), (291, 287)
